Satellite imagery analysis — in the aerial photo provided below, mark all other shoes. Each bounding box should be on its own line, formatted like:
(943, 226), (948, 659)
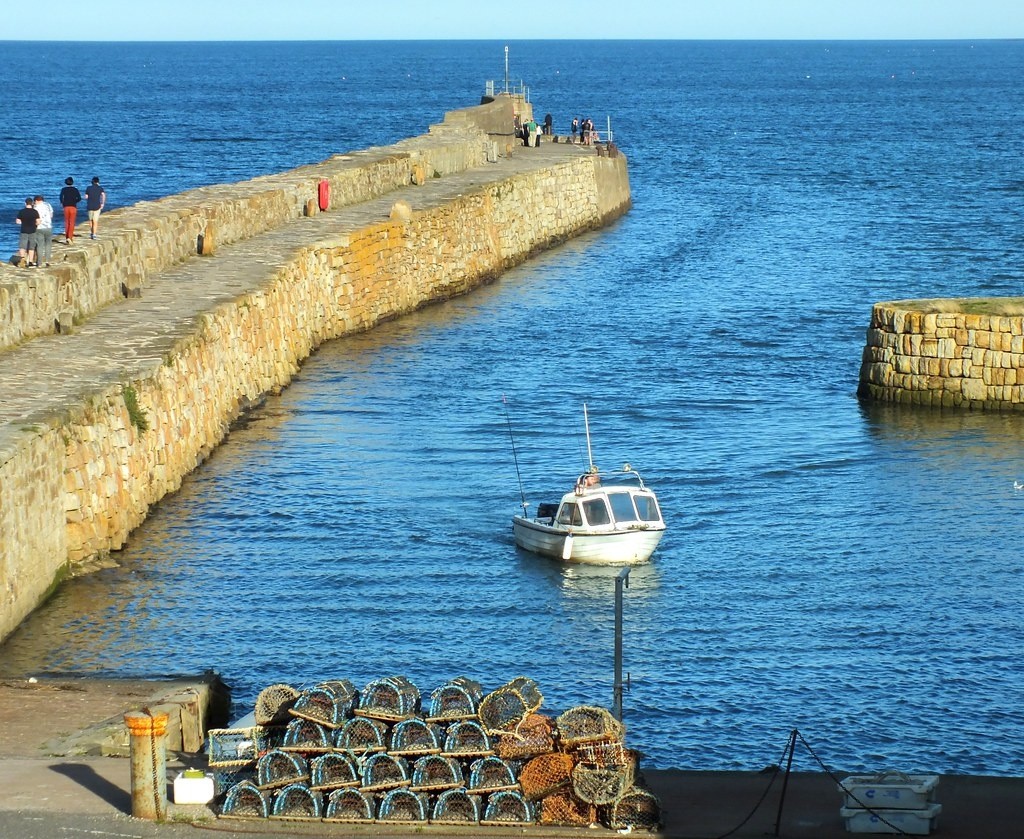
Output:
(46, 262), (49, 266)
(20, 258), (26, 269)
(90, 234), (97, 239)
(29, 262), (37, 268)
(67, 239), (73, 247)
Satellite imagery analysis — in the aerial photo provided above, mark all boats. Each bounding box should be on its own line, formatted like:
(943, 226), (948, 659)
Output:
(502, 391), (667, 568)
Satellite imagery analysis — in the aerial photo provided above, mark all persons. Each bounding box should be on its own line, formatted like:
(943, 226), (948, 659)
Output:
(582, 471), (594, 488)
(514, 115), (543, 147)
(570, 117), (594, 145)
(85, 176), (105, 239)
(543, 113), (552, 135)
(33, 196), (54, 267)
(59, 177), (81, 245)
(15, 198), (40, 269)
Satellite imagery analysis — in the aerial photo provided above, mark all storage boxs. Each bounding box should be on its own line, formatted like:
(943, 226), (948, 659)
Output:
(174, 771), (220, 805)
(208, 726), (280, 766)
